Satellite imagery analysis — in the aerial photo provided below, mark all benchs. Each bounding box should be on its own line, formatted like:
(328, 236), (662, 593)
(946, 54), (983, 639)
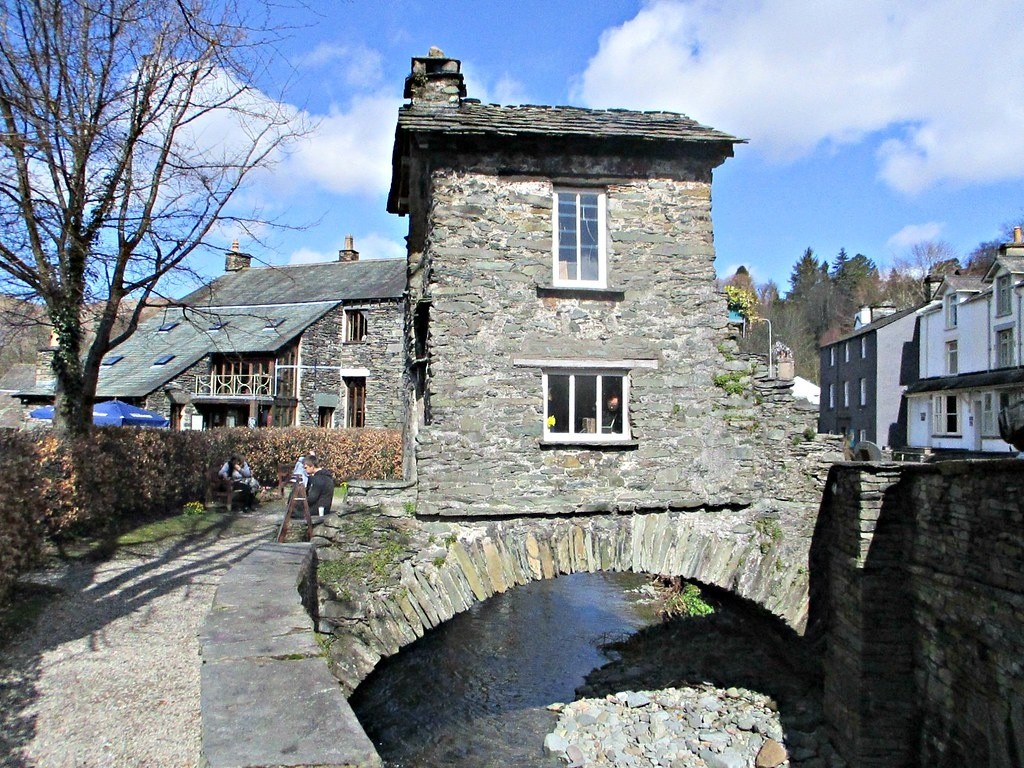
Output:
(204, 462), (247, 513)
(276, 460), (303, 498)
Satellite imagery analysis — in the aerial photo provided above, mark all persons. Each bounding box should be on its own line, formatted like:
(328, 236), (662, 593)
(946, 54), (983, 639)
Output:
(217, 453), (257, 514)
(285, 456), (335, 518)
(594, 392), (624, 434)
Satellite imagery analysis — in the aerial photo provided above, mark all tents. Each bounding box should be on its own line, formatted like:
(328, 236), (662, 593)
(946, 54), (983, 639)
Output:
(30, 397), (171, 430)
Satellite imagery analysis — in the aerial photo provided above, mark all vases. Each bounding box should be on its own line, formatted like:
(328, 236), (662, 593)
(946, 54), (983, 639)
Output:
(548, 429), (550, 432)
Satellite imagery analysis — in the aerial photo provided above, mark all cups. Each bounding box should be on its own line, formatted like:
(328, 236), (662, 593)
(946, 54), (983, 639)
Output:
(318, 506), (324, 517)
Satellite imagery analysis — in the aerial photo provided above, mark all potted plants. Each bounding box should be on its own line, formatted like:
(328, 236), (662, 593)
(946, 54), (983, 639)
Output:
(723, 285), (765, 325)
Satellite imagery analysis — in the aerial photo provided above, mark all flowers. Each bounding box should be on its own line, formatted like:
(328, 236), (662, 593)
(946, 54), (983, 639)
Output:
(548, 415), (556, 428)
